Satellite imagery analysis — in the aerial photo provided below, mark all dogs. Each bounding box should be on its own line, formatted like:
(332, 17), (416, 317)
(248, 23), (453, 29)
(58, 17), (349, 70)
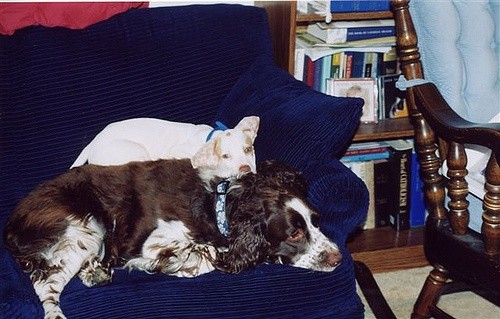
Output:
(0, 116), (343, 319)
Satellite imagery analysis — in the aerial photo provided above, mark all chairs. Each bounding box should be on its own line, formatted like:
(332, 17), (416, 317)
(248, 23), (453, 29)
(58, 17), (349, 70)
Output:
(388, 0), (500, 319)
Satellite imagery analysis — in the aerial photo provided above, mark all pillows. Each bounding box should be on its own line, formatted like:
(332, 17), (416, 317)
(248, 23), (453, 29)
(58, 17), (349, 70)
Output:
(213, 55), (365, 183)
(0, 2), (149, 35)
(437, 113), (500, 201)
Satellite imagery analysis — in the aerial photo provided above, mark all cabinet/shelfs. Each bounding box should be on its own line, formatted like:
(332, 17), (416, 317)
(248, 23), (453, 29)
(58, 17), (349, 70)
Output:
(253, 0), (430, 274)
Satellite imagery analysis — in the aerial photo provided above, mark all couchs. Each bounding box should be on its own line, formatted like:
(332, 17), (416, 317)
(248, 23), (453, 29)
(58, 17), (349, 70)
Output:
(0, 2), (371, 319)
